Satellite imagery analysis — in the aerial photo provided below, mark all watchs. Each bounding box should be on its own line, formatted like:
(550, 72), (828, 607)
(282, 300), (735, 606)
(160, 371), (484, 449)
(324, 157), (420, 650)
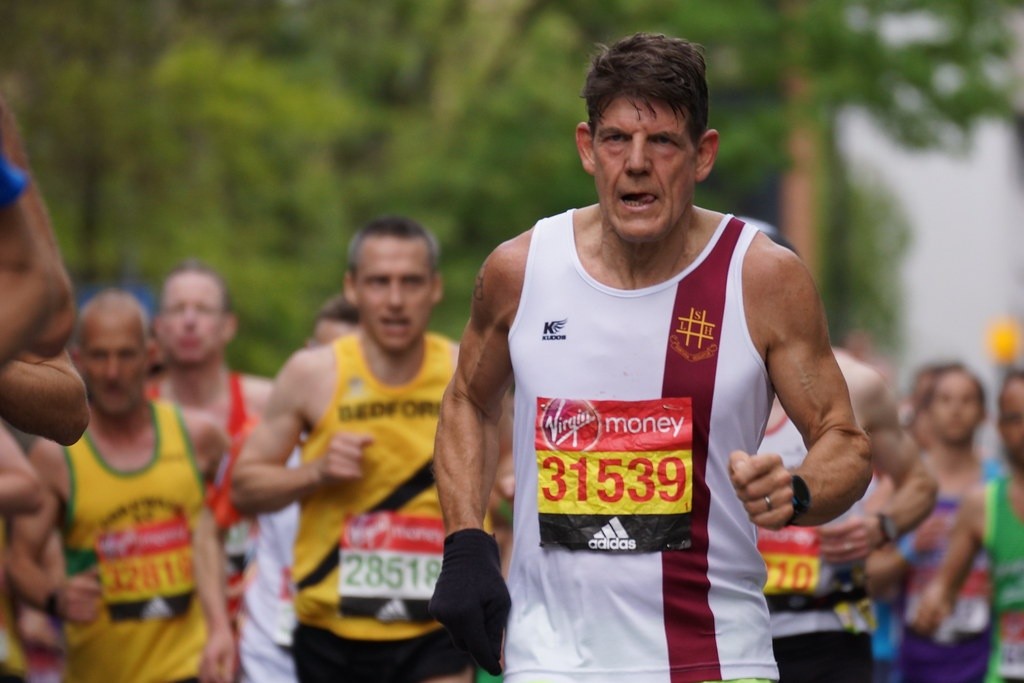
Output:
(786, 473), (810, 527)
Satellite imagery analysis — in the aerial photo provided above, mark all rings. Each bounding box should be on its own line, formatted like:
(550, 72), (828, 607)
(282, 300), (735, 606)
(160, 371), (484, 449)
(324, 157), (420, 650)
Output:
(764, 495), (774, 508)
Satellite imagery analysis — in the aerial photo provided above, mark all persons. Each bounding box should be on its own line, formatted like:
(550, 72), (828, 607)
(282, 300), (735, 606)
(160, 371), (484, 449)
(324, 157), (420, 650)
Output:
(228, 216), (513, 682)
(8, 287), (231, 682)
(432, 32), (873, 682)
(857, 361), (1024, 682)
(0, 155), (91, 682)
(148, 263), (276, 636)
(739, 218), (938, 682)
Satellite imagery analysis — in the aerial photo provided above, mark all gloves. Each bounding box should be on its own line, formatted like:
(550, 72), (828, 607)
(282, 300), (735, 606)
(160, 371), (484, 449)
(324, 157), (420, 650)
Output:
(429, 529), (511, 677)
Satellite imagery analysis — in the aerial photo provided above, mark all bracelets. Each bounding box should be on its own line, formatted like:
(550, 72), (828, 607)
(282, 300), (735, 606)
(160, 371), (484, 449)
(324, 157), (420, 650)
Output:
(43, 580), (62, 615)
(876, 511), (896, 543)
